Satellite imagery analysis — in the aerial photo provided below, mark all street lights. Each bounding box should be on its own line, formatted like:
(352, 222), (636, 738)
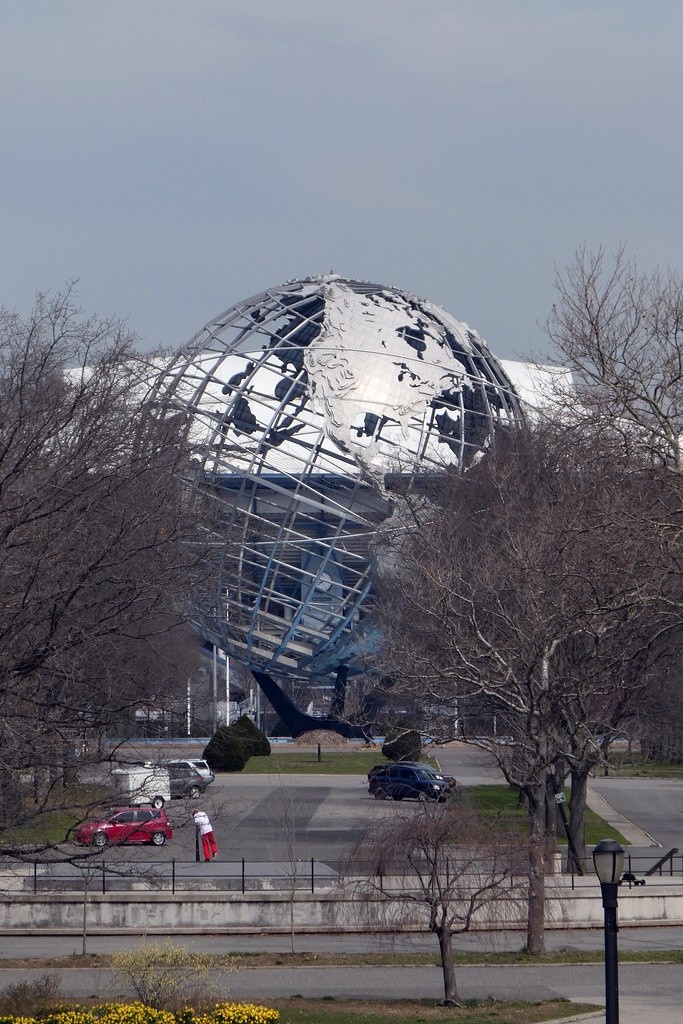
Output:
(589, 835), (630, 1024)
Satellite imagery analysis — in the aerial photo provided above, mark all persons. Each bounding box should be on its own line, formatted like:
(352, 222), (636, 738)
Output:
(191, 809), (217, 862)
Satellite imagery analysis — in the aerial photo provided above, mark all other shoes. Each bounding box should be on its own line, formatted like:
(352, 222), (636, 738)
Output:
(205, 858), (211, 862)
(212, 852), (217, 860)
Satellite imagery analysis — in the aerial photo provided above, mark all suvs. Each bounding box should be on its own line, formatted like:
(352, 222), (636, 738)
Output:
(368, 762), (458, 804)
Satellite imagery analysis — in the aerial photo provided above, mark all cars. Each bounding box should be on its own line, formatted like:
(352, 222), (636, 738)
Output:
(111, 759), (215, 809)
(73, 807), (173, 846)
